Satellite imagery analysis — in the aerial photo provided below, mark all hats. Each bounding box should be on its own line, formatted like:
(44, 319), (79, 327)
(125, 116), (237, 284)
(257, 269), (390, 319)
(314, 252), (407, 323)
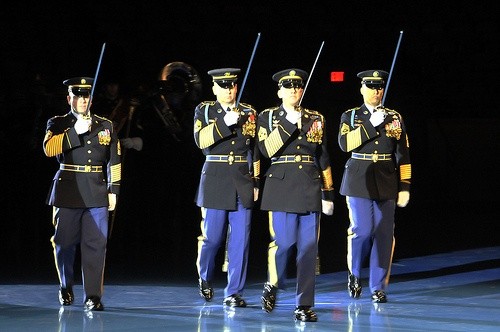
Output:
(208, 68), (241, 88)
(272, 68), (309, 88)
(357, 69), (389, 89)
(63, 77), (94, 95)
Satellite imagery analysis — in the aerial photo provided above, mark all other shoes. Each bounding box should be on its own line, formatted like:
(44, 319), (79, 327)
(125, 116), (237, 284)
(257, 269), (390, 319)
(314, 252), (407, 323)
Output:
(371, 289), (387, 303)
(200, 277), (214, 301)
(348, 270), (362, 298)
(294, 305), (318, 322)
(261, 282), (277, 312)
(84, 296), (103, 311)
(223, 293), (246, 307)
(59, 286), (74, 305)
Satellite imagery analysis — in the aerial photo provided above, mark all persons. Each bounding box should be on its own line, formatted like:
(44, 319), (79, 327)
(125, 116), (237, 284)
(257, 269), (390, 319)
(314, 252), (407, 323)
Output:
(194, 67), (259, 307)
(43, 78), (145, 311)
(254, 70), (335, 322)
(339, 71), (413, 302)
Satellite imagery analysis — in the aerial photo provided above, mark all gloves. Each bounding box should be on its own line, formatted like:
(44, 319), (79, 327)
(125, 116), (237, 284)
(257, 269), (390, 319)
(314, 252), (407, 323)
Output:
(285, 109), (301, 124)
(369, 112), (388, 127)
(397, 191), (410, 207)
(322, 199), (334, 215)
(108, 194), (116, 211)
(223, 111), (239, 126)
(74, 117), (92, 134)
(254, 187), (259, 201)
